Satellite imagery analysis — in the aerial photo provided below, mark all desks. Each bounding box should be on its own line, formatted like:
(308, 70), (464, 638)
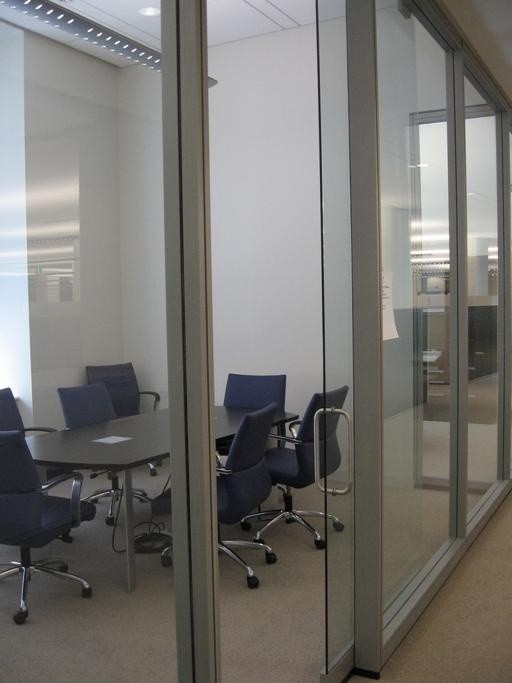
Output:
(24, 404), (300, 593)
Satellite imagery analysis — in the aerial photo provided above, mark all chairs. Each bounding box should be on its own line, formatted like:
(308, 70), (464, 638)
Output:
(59, 382), (152, 528)
(212, 372), (289, 534)
(160, 401), (281, 589)
(2, 430), (97, 623)
(83, 361), (163, 478)
(241, 384), (350, 548)
(0, 386), (80, 546)
